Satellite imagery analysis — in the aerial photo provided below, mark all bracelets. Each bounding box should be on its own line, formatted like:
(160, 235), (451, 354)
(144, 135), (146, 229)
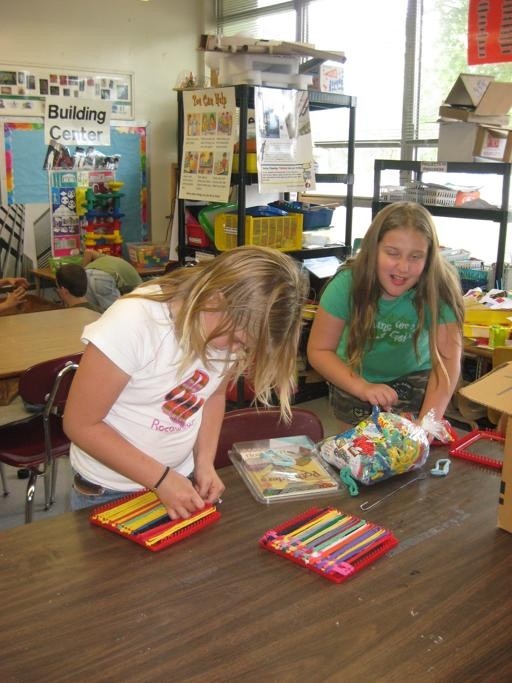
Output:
(149, 466), (171, 492)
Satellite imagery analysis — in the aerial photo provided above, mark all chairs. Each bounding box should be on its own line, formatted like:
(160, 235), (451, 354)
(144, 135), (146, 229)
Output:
(2, 351), (84, 525)
(215, 408), (323, 468)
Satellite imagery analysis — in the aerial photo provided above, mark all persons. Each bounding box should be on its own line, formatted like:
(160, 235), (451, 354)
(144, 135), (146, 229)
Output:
(80, 246), (144, 313)
(0, 276), (32, 310)
(303, 199), (468, 447)
(55, 263), (106, 316)
(61, 243), (312, 524)
(183, 111), (233, 176)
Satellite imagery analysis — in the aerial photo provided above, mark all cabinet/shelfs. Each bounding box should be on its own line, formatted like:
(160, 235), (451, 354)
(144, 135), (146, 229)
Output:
(178, 87), (356, 403)
(372, 159), (511, 422)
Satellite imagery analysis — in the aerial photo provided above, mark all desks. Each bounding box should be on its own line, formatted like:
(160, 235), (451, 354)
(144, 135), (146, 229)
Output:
(31, 268), (165, 297)
(0, 307), (103, 374)
(445, 336), (511, 426)
(2, 424), (511, 682)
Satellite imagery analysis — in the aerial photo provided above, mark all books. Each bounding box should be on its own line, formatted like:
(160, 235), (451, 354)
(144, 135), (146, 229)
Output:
(231, 434), (339, 503)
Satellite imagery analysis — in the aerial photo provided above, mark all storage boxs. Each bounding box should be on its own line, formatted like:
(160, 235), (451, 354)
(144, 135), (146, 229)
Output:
(436, 120), (511, 163)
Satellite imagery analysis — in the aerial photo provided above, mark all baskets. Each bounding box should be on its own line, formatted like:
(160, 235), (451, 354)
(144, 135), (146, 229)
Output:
(379, 184), (457, 207)
(440, 248), (487, 290)
(213, 212), (303, 251)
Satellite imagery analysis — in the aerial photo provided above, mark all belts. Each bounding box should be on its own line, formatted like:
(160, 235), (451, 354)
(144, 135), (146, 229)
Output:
(73, 472), (105, 495)
